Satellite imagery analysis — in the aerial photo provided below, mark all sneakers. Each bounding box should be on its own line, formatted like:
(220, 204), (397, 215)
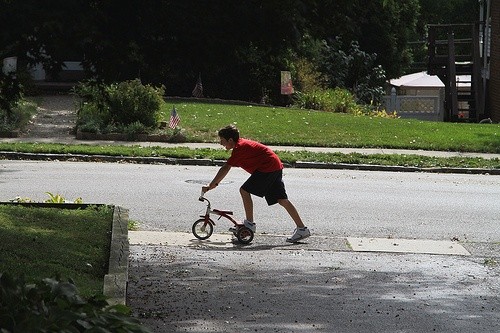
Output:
(229, 219), (256, 233)
(286, 226), (311, 242)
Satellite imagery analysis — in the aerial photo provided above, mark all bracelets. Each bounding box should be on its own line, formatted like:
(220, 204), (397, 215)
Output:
(212, 180), (218, 186)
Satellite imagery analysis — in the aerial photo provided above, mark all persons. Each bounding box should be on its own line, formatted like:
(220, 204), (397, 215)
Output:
(202, 125), (312, 243)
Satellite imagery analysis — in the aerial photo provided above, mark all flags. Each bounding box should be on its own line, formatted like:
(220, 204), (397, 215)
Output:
(193, 77), (203, 97)
(169, 107), (180, 129)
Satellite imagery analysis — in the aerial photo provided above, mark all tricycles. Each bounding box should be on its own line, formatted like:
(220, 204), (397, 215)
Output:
(192, 185), (255, 243)
